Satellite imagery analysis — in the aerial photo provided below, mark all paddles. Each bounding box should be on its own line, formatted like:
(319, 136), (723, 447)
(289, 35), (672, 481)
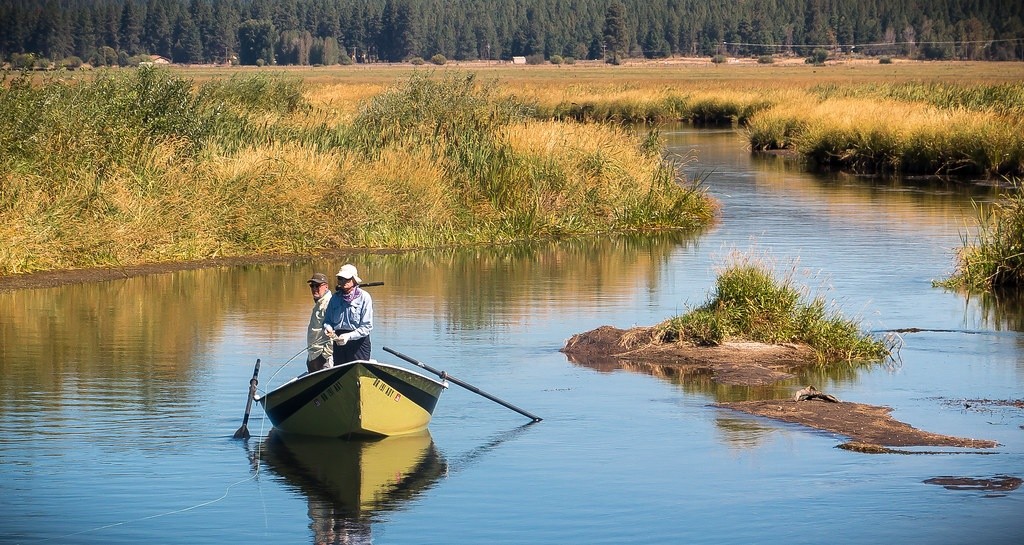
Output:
(232, 358), (261, 439)
(383, 346), (544, 421)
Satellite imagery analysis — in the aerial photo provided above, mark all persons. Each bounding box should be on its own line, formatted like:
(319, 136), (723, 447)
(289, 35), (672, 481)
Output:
(302, 272), (335, 370)
(322, 263), (373, 364)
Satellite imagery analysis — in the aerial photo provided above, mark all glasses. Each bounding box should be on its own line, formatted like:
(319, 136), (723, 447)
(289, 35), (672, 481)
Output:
(338, 276), (353, 283)
(310, 284), (327, 287)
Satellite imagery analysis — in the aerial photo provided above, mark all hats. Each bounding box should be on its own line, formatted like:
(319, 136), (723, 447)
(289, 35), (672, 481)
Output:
(336, 264), (359, 279)
(307, 273), (328, 283)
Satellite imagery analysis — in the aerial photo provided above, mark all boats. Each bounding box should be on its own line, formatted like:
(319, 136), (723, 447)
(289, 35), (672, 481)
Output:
(230, 345), (543, 440)
